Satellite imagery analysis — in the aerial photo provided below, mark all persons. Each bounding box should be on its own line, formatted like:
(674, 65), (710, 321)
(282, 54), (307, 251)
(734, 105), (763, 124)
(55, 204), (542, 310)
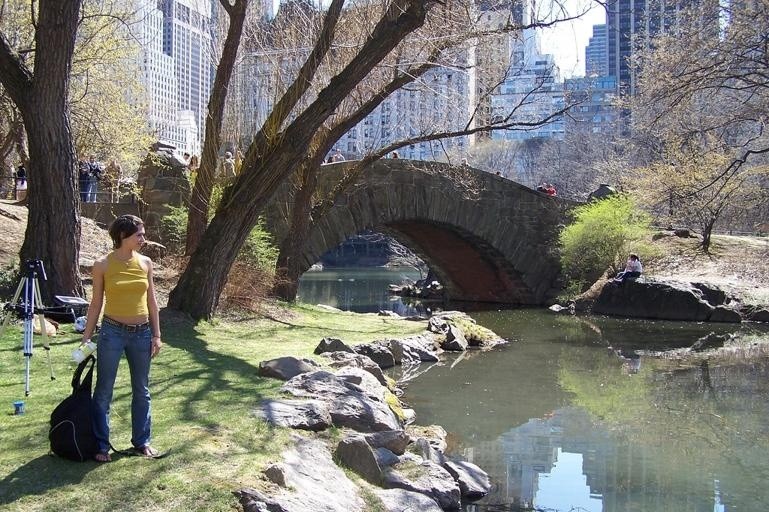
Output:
(539, 182), (548, 193)
(103, 158), (124, 203)
(614, 254), (642, 285)
(222, 152), (237, 177)
(78, 213), (164, 464)
(496, 171), (501, 176)
(181, 152), (190, 162)
(0, 157), (15, 189)
(460, 157), (470, 166)
(16, 160), (26, 189)
(391, 152), (398, 159)
(327, 157), (335, 163)
(613, 348), (642, 374)
(546, 184), (556, 196)
(188, 154), (201, 171)
(87, 154), (102, 203)
(334, 148), (345, 161)
(605, 257), (635, 285)
(76, 152), (91, 202)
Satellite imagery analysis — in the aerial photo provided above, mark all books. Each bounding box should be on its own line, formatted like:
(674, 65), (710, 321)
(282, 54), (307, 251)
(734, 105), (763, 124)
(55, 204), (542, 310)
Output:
(71, 339), (98, 364)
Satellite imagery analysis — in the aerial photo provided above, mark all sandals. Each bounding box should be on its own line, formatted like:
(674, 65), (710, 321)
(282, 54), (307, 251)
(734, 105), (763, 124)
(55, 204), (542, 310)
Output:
(93, 453), (112, 462)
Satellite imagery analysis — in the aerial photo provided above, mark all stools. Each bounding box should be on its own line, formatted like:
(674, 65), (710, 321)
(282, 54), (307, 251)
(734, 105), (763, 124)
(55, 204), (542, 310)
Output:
(53, 295), (89, 323)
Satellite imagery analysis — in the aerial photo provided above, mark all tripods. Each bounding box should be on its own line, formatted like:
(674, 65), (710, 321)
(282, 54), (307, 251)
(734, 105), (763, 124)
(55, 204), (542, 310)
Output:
(0, 259), (55, 396)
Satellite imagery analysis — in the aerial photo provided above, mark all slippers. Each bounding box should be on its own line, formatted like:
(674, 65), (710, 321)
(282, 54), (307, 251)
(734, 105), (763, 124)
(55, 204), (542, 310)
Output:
(134, 443), (156, 456)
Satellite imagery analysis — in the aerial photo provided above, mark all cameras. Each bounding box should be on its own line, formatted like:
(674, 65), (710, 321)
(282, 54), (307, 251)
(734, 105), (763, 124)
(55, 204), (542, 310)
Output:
(94, 167), (102, 173)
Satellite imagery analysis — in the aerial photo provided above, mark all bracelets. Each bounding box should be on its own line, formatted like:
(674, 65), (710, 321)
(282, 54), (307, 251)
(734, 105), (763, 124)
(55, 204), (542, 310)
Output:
(152, 335), (161, 339)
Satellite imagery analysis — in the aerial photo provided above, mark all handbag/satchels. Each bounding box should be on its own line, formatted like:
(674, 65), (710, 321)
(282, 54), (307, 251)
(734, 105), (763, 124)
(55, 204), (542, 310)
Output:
(48, 354), (96, 463)
